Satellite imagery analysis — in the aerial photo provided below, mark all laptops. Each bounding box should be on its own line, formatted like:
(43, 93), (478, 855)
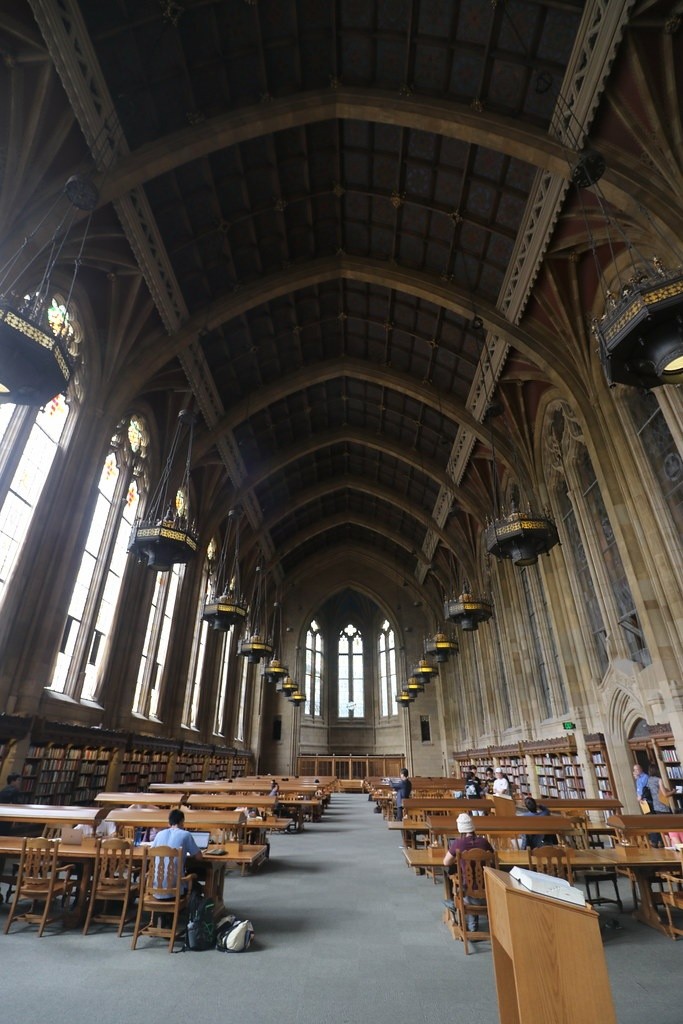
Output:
(449, 838), (455, 849)
(62, 828), (83, 844)
(188, 831), (210, 853)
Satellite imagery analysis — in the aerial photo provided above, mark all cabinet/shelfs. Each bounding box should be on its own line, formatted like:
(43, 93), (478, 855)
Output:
(0, 711), (256, 837)
(452, 723), (683, 823)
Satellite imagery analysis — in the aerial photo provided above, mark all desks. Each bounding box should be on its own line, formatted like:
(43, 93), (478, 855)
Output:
(0, 776), (338, 917)
(365, 772), (683, 938)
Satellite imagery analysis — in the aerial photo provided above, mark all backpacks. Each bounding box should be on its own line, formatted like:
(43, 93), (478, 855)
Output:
(184, 893), (215, 944)
(639, 786), (654, 815)
(214, 920), (255, 953)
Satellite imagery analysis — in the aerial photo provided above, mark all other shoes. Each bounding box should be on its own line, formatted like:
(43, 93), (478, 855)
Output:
(442, 898), (456, 913)
(467, 922), (477, 931)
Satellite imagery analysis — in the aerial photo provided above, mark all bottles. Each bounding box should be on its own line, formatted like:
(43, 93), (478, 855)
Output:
(134, 827), (142, 847)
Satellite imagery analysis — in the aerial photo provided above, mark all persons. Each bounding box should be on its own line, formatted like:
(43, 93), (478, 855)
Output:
(443, 813), (494, 942)
(269, 783), (294, 834)
(519, 797), (559, 845)
(465, 765), (486, 816)
(485, 768), (509, 795)
(228, 779), (233, 783)
(233, 807), (270, 865)
(633, 763), (678, 848)
(0, 772), (24, 902)
(257, 775), (319, 783)
(388, 768), (412, 848)
(72, 821), (116, 909)
(150, 809), (203, 941)
(669, 807), (683, 852)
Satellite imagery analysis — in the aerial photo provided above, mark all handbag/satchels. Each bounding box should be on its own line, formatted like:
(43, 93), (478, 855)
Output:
(213, 914), (236, 933)
(187, 921), (211, 951)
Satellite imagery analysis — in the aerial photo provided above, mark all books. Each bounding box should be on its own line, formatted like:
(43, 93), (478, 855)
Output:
(535, 754), (613, 822)
(175, 756), (203, 781)
(232, 760), (245, 777)
(93, 750), (112, 806)
(210, 758), (228, 779)
(20, 746), (97, 805)
(459, 760), (493, 778)
(509, 866), (586, 907)
(118, 753), (149, 792)
(499, 758), (531, 798)
(149, 754), (169, 782)
(662, 750), (683, 794)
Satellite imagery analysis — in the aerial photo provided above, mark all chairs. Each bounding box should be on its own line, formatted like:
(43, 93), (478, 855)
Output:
(2, 791), (298, 953)
(364, 793), (683, 955)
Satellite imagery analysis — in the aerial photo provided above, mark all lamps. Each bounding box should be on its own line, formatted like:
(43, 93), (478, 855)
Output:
(1, 49), (683, 707)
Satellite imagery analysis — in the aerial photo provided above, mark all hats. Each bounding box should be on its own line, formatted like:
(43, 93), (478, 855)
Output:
(495, 768), (502, 772)
(456, 813), (475, 833)
